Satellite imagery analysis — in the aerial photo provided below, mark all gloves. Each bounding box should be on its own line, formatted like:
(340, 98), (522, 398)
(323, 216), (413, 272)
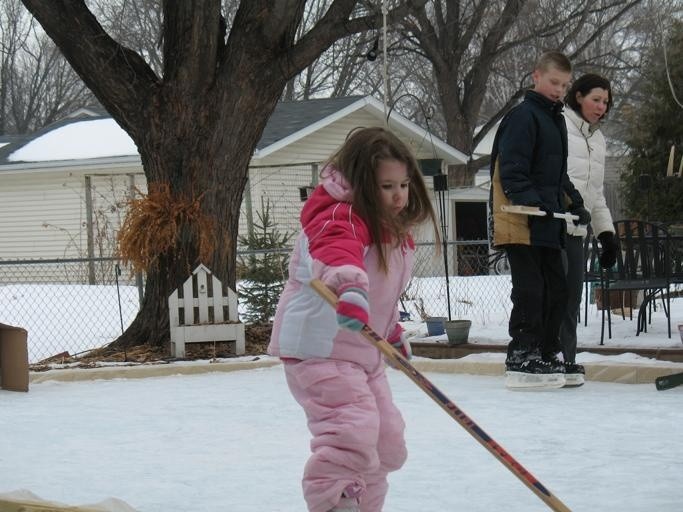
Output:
(600, 232), (616, 269)
(532, 206), (553, 224)
(336, 287), (369, 334)
(386, 323), (411, 359)
(569, 206), (591, 225)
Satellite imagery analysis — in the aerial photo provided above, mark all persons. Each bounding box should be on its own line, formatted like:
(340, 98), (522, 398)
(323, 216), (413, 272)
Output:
(562, 73), (616, 364)
(268, 126), (443, 511)
(488, 51), (585, 389)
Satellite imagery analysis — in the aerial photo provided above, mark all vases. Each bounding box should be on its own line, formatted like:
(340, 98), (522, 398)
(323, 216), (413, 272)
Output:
(426, 317), (471, 345)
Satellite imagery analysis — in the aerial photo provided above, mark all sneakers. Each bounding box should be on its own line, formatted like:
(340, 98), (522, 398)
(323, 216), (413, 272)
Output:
(506, 339), (584, 375)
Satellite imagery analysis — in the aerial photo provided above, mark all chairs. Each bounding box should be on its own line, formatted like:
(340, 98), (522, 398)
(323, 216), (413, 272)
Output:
(577, 220), (683, 345)
